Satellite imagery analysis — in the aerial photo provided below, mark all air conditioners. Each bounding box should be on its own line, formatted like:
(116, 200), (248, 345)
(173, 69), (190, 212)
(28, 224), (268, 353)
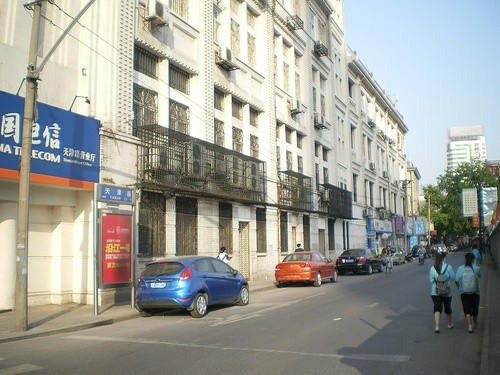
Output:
(315, 39), (327, 55)
(371, 162), (376, 170)
(314, 115), (326, 128)
(218, 46), (235, 63)
(383, 171), (388, 177)
(364, 209), (369, 215)
(290, 98), (300, 111)
(322, 190), (332, 201)
(223, 156), (241, 185)
(144, 0), (170, 27)
(182, 141), (205, 178)
(149, 148), (172, 169)
(242, 162), (259, 189)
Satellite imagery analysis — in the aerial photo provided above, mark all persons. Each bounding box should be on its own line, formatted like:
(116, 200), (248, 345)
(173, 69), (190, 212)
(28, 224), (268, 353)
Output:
(294, 243), (305, 261)
(471, 244), (483, 265)
(383, 245), (397, 271)
(416, 245), (427, 259)
(454, 253), (482, 333)
(217, 247), (233, 265)
(430, 252), (456, 333)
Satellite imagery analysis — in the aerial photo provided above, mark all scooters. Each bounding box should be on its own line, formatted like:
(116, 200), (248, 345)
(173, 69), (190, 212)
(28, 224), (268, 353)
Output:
(417, 253), (425, 264)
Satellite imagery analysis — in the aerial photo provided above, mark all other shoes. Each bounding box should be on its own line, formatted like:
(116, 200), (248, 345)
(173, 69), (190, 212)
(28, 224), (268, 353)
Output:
(468, 325), (473, 332)
(434, 328), (440, 333)
(448, 323), (455, 328)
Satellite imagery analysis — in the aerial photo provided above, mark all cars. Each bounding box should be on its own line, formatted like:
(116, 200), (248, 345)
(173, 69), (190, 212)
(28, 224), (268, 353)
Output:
(274, 251), (337, 287)
(411, 245), (428, 258)
(431, 243), (450, 256)
(448, 244), (458, 251)
(381, 246), (408, 265)
(134, 255), (249, 318)
(335, 248), (384, 276)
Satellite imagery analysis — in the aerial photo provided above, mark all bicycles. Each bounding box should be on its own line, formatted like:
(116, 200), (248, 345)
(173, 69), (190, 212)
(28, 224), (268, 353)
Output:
(384, 258), (394, 274)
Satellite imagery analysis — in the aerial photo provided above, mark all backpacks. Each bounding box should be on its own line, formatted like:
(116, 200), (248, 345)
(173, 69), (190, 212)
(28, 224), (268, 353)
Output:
(461, 267), (478, 293)
(433, 264), (451, 295)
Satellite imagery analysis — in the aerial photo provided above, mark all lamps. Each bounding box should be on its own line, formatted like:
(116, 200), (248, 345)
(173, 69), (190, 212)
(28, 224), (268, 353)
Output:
(69, 95), (91, 112)
